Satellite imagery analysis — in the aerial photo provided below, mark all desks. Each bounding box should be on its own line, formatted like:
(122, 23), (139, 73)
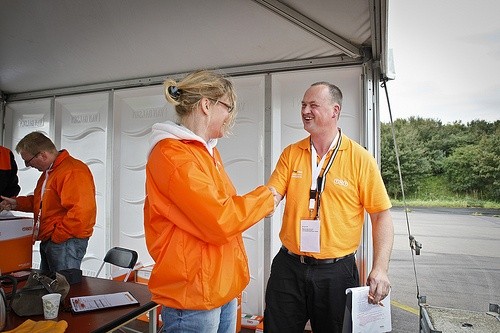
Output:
(0, 268), (161, 333)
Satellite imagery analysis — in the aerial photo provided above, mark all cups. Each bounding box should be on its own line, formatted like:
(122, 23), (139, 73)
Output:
(42, 293), (62, 319)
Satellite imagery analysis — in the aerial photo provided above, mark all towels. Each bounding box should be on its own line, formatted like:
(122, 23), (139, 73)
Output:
(0, 318), (68, 333)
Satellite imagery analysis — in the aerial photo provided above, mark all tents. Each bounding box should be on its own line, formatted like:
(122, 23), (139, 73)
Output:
(0, 0), (394, 333)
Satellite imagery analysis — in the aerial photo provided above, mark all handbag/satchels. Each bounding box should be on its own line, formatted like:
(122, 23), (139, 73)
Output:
(11, 271), (70, 316)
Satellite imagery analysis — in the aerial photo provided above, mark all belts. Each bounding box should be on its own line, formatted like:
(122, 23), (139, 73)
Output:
(281, 245), (357, 267)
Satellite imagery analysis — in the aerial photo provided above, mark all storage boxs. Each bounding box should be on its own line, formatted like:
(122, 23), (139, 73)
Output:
(0, 216), (36, 274)
(240, 313), (264, 328)
(254, 321), (263, 333)
(235, 291), (242, 333)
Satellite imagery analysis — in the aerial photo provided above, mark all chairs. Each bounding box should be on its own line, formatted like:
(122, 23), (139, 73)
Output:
(95, 246), (139, 283)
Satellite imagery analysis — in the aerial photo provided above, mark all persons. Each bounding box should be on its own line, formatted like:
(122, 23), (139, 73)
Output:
(262, 81), (394, 333)
(144, 70), (278, 333)
(0, 132), (96, 272)
(0, 145), (21, 203)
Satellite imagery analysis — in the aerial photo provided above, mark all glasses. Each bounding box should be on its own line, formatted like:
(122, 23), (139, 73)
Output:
(24, 152), (41, 167)
(206, 97), (234, 113)
